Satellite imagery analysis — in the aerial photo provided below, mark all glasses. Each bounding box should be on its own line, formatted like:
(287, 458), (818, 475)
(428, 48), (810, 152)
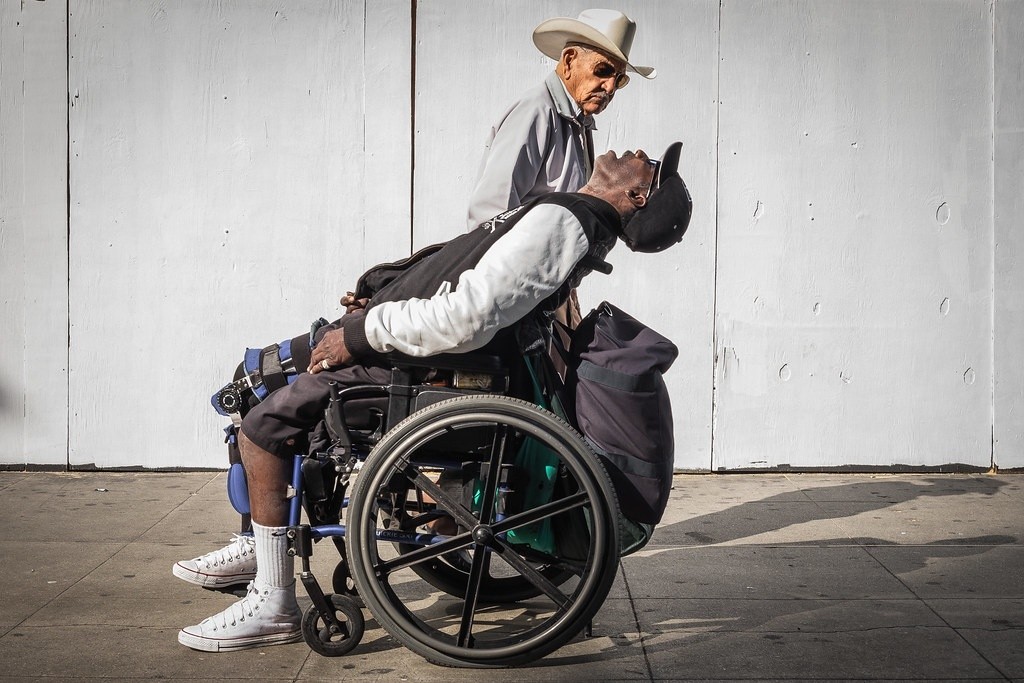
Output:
(646, 158), (661, 199)
(573, 44), (630, 89)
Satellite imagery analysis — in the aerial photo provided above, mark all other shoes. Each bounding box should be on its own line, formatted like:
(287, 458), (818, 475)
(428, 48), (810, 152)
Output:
(410, 492), (457, 536)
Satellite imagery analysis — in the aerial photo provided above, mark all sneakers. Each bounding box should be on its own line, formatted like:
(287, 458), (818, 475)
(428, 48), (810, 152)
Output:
(172, 532), (257, 587)
(177, 576), (304, 652)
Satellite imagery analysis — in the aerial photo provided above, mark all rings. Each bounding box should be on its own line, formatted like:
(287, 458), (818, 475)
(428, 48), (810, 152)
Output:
(322, 361), (330, 370)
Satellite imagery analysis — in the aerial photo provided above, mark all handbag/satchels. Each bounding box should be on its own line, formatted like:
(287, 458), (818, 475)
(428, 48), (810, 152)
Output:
(500, 300), (679, 562)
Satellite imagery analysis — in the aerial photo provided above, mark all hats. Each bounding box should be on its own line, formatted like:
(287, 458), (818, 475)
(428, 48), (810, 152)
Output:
(532, 9), (657, 79)
(619, 142), (693, 254)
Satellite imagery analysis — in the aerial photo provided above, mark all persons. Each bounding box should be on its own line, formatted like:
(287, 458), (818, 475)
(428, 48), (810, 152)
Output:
(414, 6), (656, 541)
(173, 141), (694, 653)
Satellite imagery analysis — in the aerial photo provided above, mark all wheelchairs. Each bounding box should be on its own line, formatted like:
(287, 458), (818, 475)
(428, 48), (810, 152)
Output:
(202, 257), (623, 670)
